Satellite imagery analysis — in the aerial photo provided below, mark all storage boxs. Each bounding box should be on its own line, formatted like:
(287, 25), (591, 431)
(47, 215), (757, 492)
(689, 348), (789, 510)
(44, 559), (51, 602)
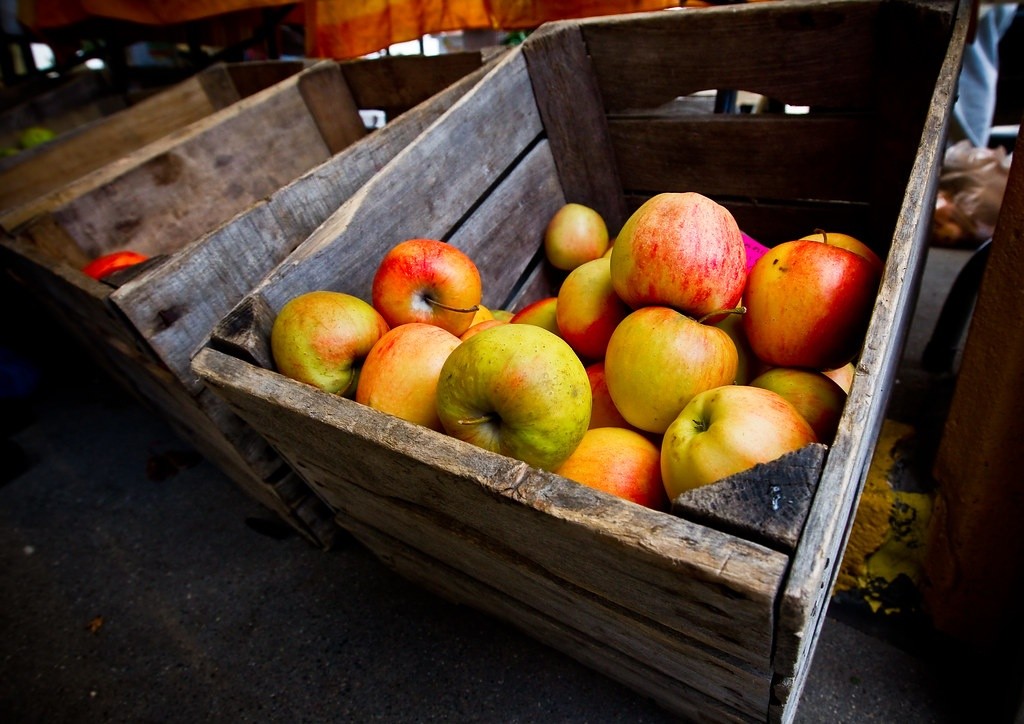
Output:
(0, 0), (977, 724)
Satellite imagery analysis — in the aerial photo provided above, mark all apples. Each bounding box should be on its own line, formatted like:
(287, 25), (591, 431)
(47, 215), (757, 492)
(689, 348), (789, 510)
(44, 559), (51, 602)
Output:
(271, 194), (883, 507)
(79, 251), (150, 279)
(0, 128), (54, 160)
